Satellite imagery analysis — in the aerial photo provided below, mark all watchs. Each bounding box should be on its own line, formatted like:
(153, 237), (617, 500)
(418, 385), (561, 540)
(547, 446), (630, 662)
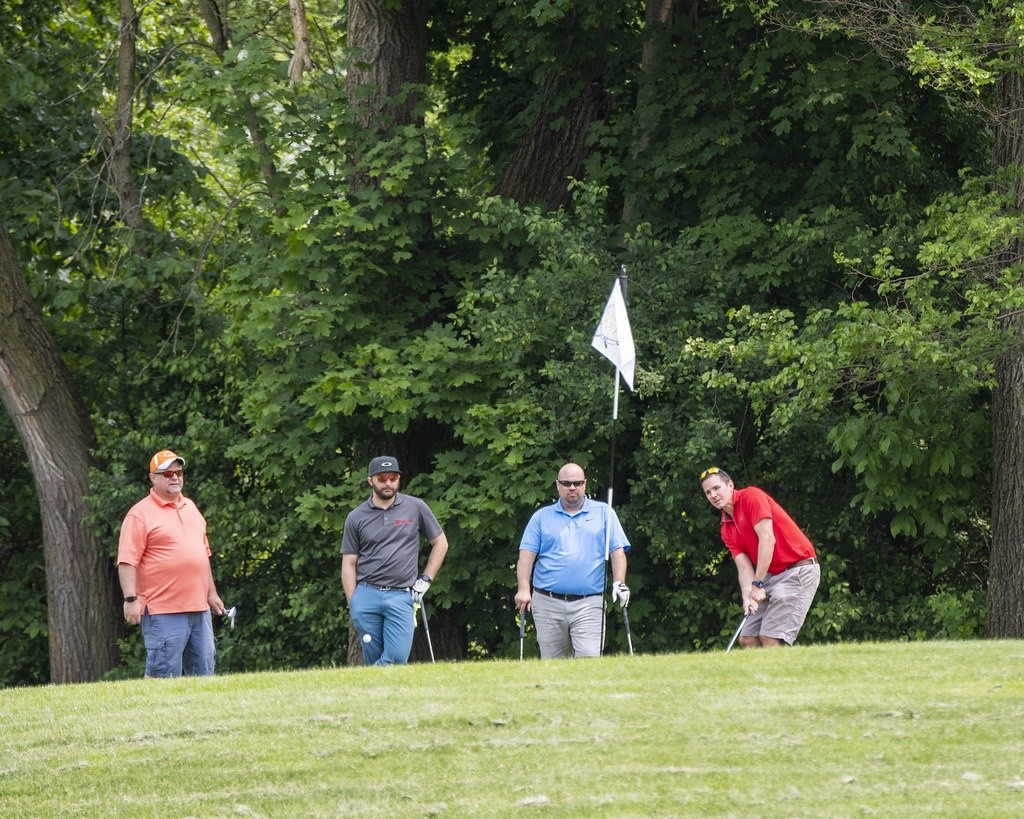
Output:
(752, 580), (765, 588)
(421, 574), (432, 582)
(123, 596), (137, 602)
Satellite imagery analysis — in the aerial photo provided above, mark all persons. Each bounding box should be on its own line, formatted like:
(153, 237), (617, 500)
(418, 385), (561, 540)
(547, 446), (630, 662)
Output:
(117, 450), (225, 678)
(515, 463), (632, 660)
(340, 456), (448, 666)
(699, 466), (820, 648)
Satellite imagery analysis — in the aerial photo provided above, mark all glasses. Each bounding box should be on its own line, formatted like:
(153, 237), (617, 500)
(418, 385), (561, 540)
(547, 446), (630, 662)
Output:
(154, 470), (184, 477)
(700, 467), (730, 481)
(558, 480), (585, 487)
(372, 474), (399, 482)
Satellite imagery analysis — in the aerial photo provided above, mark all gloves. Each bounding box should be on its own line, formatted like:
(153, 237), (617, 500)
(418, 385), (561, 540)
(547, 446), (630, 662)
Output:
(612, 581), (630, 607)
(411, 578), (430, 604)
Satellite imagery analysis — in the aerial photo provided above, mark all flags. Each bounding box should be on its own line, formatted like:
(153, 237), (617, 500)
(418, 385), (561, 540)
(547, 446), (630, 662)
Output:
(591, 270), (638, 392)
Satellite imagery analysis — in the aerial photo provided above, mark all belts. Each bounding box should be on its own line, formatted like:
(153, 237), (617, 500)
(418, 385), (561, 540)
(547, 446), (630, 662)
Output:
(784, 559), (818, 571)
(360, 580), (410, 591)
(535, 587), (601, 601)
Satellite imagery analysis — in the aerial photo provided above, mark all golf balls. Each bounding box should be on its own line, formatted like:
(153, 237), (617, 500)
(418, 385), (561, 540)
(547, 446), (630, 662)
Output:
(363, 634), (371, 643)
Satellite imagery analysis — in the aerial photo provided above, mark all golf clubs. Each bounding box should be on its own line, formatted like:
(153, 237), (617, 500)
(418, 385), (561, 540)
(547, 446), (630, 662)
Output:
(420, 598), (435, 663)
(724, 609), (752, 653)
(519, 609), (525, 660)
(624, 606), (634, 655)
(221, 606), (237, 619)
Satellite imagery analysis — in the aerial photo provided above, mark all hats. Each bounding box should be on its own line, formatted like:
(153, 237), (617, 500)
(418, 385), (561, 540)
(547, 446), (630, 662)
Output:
(368, 456), (402, 476)
(150, 450), (185, 473)
